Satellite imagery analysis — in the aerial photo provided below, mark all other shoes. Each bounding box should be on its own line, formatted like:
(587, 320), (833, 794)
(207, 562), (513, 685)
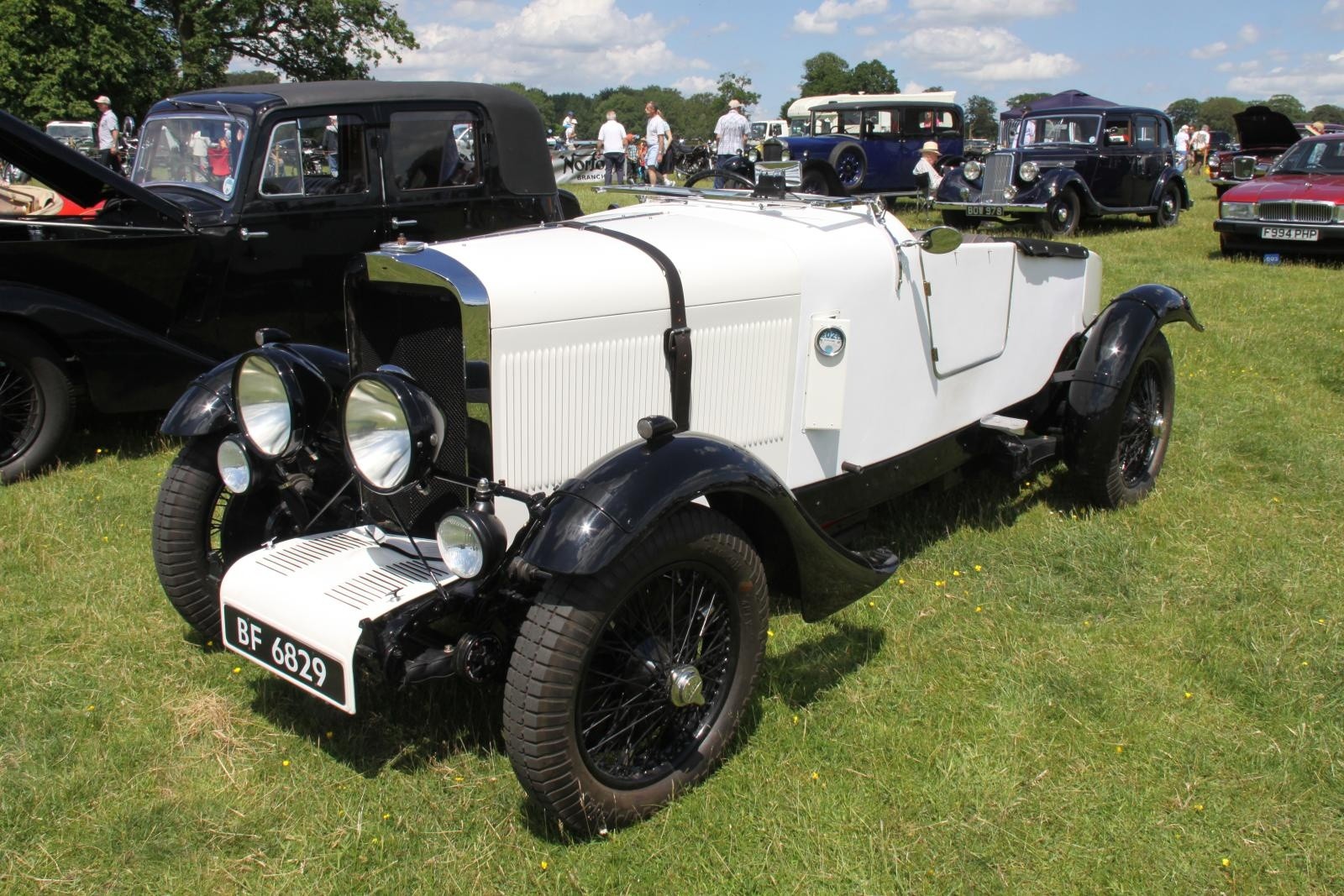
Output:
(670, 181), (676, 187)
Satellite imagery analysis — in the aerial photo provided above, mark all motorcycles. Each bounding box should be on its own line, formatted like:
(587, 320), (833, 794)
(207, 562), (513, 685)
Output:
(301, 140), (323, 175)
(671, 137), (718, 179)
(266, 144), (288, 178)
(117, 116), (139, 179)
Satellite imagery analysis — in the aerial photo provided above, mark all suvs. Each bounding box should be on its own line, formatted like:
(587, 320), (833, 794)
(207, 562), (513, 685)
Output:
(722, 91), (966, 209)
(0, 80), (585, 486)
(927, 89), (1194, 239)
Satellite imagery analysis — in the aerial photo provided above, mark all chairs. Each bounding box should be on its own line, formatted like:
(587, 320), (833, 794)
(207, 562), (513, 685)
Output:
(284, 174), (339, 197)
(1322, 155), (1344, 170)
(394, 128), (467, 188)
(911, 171), (932, 222)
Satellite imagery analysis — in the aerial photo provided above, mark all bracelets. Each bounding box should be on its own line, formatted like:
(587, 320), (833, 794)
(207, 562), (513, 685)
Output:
(659, 151), (663, 154)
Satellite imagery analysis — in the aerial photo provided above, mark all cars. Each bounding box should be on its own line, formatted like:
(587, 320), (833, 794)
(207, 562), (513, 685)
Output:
(452, 124), (475, 161)
(1207, 105), (1344, 198)
(272, 138), (320, 167)
(1109, 133), (1129, 142)
(46, 121), (99, 158)
(1209, 131), (1232, 156)
(1213, 133), (1344, 259)
(152, 161), (1205, 840)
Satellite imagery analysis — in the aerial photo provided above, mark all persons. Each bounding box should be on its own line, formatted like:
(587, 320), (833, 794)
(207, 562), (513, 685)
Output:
(656, 110), (674, 178)
(191, 123), (243, 181)
(95, 96), (127, 178)
(562, 111), (577, 150)
(598, 111), (628, 186)
(626, 133), (641, 184)
(913, 141), (943, 199)
(637, 142), (648, 179)
(924, 112), (939, 127)
(1175, 125), (1225, 176)
(272, 148), (284, 177)
(1012, 120), (1036, 153)
(1302, 122), (1323, 169)
(323, 116), (338, 177)
(714, 99), (751, 189)
(645, 101), (676, 187)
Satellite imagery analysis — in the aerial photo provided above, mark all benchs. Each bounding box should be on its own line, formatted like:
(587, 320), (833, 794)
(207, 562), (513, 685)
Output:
(907, 225), (994, 245)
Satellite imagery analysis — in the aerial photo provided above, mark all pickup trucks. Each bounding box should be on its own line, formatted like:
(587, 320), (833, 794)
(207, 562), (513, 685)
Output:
(745, 120), (802, 152)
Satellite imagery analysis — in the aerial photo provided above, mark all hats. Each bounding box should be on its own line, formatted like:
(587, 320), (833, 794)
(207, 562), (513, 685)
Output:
(728, 99), (745, 108)
(1181, 124), (1189, 130)
(571, 119), (578, 124)
(1305, 121), (1326, 136)
(918, 141), (943, 156)
(568, 111), (574, 115)
(94, 96), (111, 105)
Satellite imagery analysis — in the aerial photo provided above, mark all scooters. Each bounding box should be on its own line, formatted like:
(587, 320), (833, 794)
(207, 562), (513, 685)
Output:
(547, 128), (568, 152)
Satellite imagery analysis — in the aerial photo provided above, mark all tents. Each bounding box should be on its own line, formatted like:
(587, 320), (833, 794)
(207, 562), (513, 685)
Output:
(998, 89), (1146, 147)
